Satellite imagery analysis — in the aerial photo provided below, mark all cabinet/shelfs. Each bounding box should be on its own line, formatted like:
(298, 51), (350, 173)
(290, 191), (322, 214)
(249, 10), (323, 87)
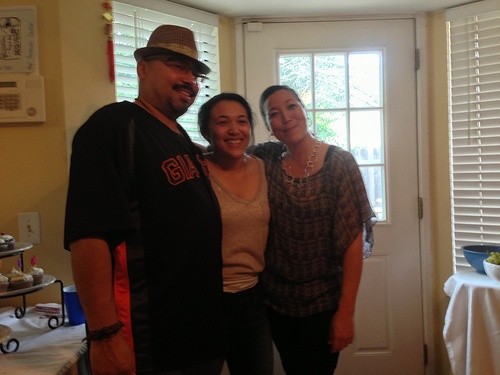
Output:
(0, 234), (64, 354)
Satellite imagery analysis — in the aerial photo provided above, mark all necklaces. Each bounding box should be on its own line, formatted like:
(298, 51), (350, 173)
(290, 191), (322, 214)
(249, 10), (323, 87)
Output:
(275, 139), (331, 195)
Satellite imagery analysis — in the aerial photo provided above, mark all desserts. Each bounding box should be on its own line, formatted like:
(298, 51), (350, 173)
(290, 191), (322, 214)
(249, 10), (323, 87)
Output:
(0, 266), (44, 292)
(0, 233), (15, 252)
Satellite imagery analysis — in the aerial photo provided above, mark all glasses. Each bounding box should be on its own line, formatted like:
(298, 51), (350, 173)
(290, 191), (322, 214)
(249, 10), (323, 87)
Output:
(144, 56), (208, 86)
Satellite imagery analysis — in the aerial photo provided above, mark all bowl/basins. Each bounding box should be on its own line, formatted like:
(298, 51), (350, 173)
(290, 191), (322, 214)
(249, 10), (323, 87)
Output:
(461, 245), (500, 274)
(483, 259), (500, 283)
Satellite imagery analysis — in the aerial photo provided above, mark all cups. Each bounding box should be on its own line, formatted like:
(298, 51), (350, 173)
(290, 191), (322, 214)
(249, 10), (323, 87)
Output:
(62, 285), (86, 326)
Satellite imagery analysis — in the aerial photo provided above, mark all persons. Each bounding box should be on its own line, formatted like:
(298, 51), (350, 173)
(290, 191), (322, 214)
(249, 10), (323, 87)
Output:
(191, 85), (377, 375)
(197, 93), (276, 374)
(64, 24), (222, 374)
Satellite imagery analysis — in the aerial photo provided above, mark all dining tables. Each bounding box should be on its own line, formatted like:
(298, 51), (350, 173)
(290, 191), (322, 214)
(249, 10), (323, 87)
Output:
(0, 302), (87, 375)
(443, 272), (500, 375)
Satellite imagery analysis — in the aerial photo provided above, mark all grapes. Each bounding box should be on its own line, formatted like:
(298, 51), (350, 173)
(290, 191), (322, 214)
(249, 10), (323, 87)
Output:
(485, 249), (500, 265)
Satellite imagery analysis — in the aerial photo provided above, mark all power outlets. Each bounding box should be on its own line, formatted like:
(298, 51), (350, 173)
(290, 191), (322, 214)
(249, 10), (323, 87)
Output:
(17, 212), (40, 244)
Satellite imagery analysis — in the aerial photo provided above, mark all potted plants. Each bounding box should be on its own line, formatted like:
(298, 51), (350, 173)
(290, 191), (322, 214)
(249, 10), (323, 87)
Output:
(484, 252), (500, 277)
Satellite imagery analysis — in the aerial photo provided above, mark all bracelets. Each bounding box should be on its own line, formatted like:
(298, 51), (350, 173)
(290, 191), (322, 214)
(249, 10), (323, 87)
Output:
(86, 320), (124, 342)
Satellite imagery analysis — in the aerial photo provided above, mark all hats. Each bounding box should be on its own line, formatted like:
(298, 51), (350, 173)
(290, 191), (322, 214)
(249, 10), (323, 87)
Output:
(134, 25), (211, 75)
